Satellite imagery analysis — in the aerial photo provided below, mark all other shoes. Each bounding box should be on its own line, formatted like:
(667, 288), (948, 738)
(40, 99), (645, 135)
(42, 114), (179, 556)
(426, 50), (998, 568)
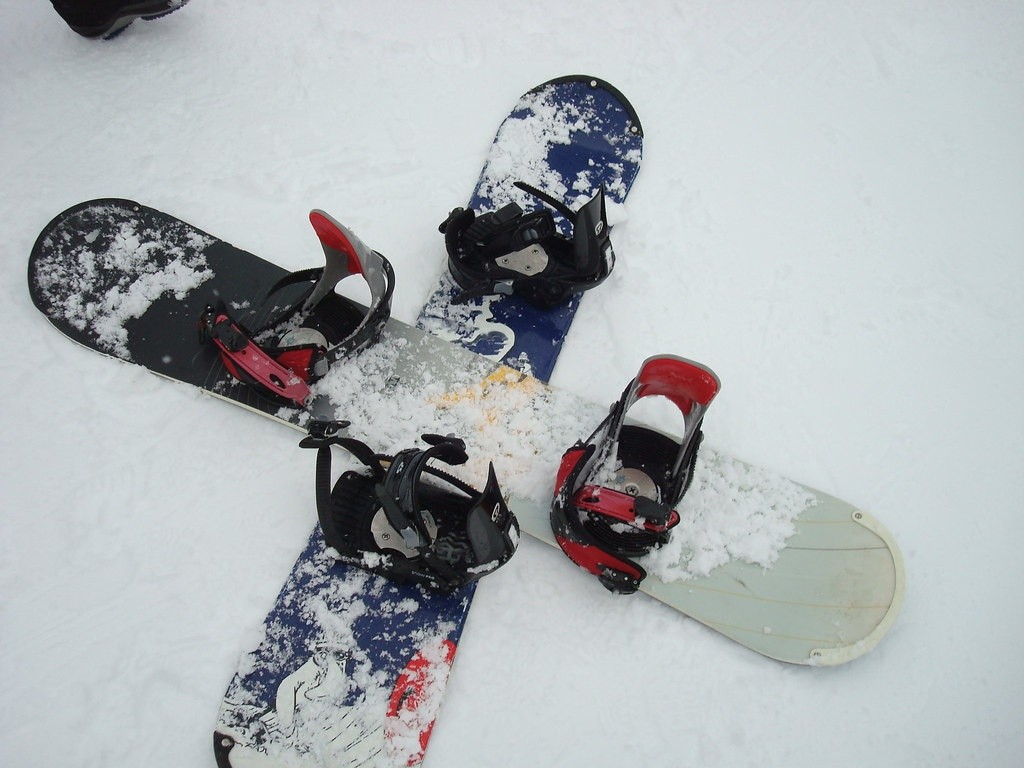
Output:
(51, 0), (188, 41)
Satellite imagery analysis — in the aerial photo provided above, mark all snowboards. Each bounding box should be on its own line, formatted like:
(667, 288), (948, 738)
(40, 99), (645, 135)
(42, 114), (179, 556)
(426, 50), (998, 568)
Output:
(213, 75), (644, 768)
(25, 196), (906, 669)
